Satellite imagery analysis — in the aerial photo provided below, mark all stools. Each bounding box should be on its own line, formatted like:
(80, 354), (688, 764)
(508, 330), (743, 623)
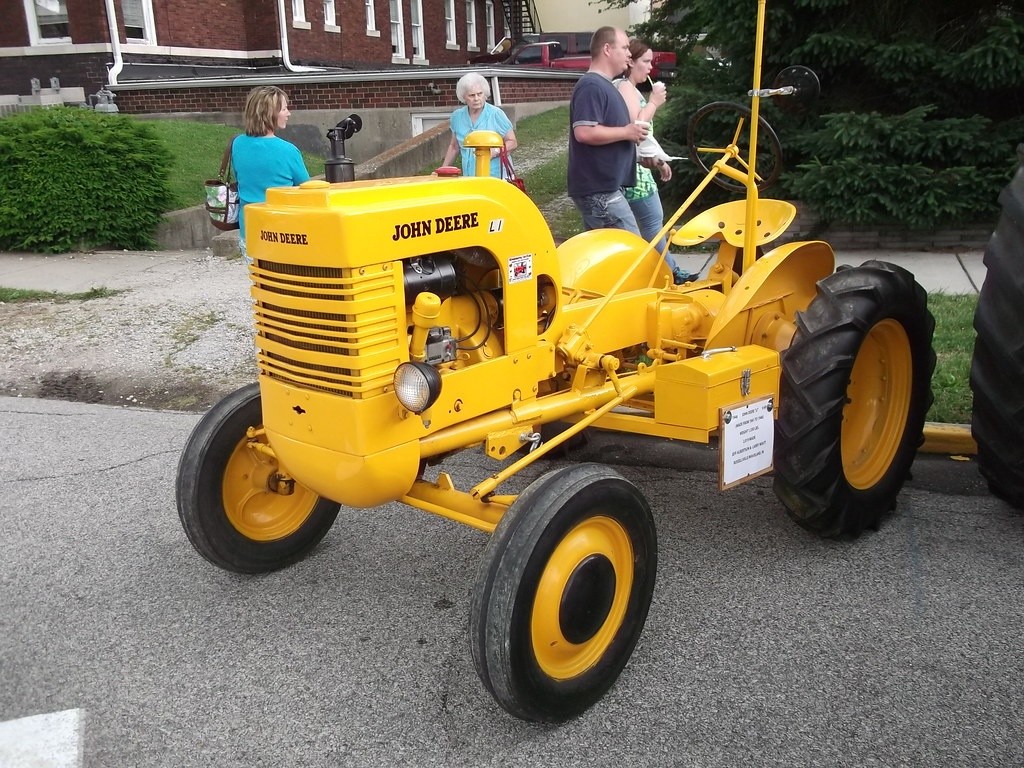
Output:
(671, 197), (796, 281)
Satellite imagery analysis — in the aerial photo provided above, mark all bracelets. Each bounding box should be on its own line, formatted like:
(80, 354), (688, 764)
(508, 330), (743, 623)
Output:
(649, 101), (657, 108)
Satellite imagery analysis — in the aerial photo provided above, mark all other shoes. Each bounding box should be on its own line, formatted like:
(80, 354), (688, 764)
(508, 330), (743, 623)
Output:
(672, 268), (698, 285)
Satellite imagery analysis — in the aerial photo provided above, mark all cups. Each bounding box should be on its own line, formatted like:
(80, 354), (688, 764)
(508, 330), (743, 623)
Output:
(635, 120), (650, 128)
(652, 82), (665, 93)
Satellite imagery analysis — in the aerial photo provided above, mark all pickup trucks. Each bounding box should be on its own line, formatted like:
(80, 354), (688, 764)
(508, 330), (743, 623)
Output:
(493, 42), (676, 91)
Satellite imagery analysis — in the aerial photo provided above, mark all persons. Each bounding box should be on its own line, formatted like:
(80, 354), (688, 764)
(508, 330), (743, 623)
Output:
(431, 72), (526, 193)
(230, 85), (309, 268)
(567, 26), (699, 285)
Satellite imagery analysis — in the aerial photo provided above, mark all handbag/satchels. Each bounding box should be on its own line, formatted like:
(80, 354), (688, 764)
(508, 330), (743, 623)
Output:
(497, 144), (529, 198)
(204, 135), (239, 230)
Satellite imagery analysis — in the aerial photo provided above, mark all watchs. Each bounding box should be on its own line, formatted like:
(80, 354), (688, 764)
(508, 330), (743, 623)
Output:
(494, 148), (499, 157)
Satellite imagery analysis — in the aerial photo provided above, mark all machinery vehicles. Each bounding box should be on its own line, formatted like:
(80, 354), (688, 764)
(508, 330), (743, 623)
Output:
(178, 0), (941, 726)
